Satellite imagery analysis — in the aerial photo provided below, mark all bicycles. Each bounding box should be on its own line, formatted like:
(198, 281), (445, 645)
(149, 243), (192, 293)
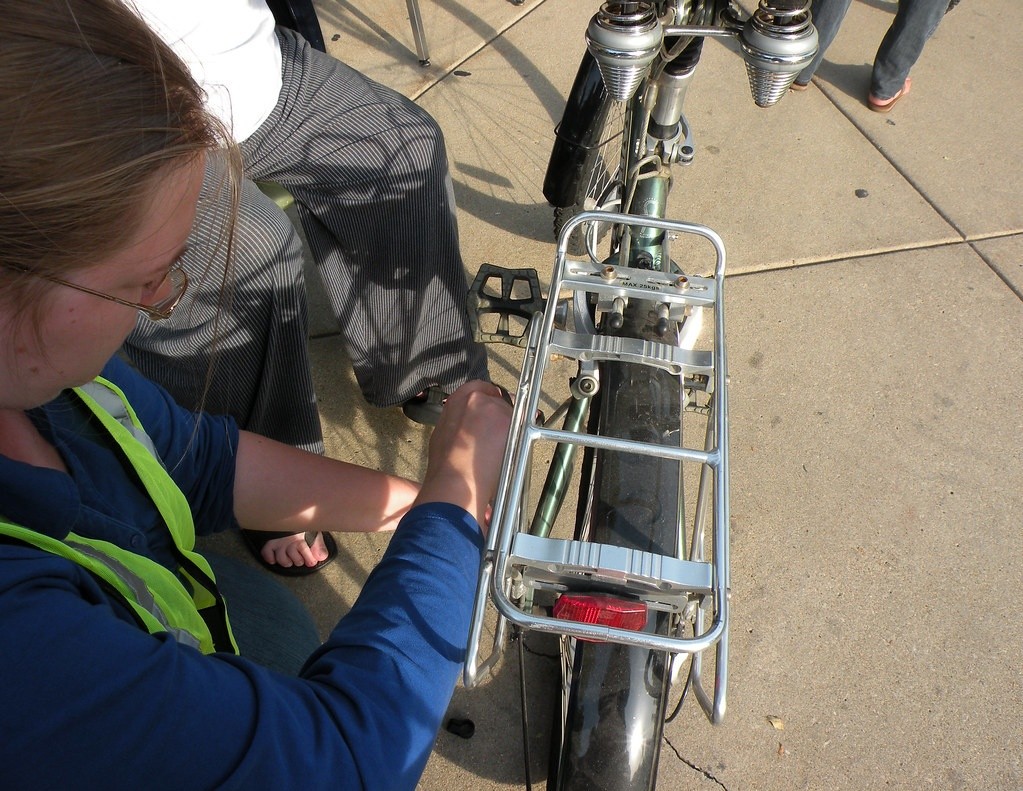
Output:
(443, 0), (826, 791)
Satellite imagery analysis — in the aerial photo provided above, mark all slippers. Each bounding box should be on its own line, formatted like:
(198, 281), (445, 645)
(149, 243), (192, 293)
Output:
(867, 79), (905, 114)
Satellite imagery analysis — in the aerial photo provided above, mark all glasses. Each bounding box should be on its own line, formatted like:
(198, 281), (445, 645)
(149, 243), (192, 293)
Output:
(1, 256), (190, 321)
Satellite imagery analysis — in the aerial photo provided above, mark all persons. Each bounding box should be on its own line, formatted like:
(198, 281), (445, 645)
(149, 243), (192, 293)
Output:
(67, 0), (547, 579)
(1, 0), (525, 791)
(785, 0), (959, 113)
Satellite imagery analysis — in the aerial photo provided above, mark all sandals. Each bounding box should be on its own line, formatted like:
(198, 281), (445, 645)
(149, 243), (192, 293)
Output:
(236, 527), (340, 577)
(402, 381), (545, 442)
